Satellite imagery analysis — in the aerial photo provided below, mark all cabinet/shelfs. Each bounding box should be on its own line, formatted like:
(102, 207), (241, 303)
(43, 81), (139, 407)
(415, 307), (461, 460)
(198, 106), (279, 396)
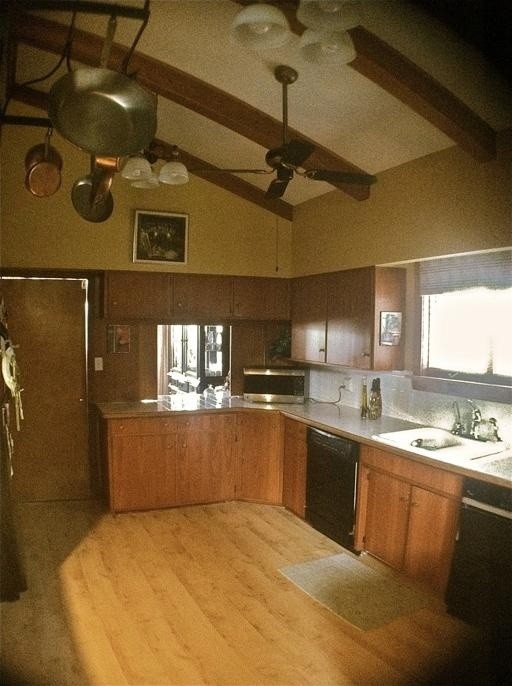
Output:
(98, 269), (290, 324)
(106, 324), (132, 355)
(236, 411), (307, 521)
(88, 409), (240, 515)
(353, 442), (464, 596)
(290, 266), (406, 371)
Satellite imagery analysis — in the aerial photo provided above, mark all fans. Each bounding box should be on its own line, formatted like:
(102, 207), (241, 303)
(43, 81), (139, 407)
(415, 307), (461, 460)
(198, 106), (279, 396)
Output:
(187, 62), (378, 202)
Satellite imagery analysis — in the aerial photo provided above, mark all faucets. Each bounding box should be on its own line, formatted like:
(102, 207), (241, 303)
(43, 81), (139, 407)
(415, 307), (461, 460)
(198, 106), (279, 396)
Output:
(451, 400), (462, 436)
(463, 397), (481, 439)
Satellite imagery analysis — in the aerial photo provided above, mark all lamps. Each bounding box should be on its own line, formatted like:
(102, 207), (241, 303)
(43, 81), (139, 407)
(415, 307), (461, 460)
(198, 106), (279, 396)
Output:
(121, 156), (189, 191)
(228, 0), (369, 69)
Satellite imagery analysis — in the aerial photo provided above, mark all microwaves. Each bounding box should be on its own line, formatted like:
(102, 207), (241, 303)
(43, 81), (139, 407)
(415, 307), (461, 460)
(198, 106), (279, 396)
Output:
(242, 366), (310, 404)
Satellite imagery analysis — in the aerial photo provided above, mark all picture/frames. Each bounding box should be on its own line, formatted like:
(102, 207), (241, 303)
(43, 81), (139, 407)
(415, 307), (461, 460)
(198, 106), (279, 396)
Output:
(131, 209), (191, 266)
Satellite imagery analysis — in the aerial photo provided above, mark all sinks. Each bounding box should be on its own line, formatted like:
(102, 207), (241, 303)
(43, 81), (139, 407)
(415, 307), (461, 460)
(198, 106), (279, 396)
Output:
(406, 435), (461, 450)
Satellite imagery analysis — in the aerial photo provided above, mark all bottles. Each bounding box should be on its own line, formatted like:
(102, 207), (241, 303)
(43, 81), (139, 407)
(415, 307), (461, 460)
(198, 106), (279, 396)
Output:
(358, 376), (382, 421)
